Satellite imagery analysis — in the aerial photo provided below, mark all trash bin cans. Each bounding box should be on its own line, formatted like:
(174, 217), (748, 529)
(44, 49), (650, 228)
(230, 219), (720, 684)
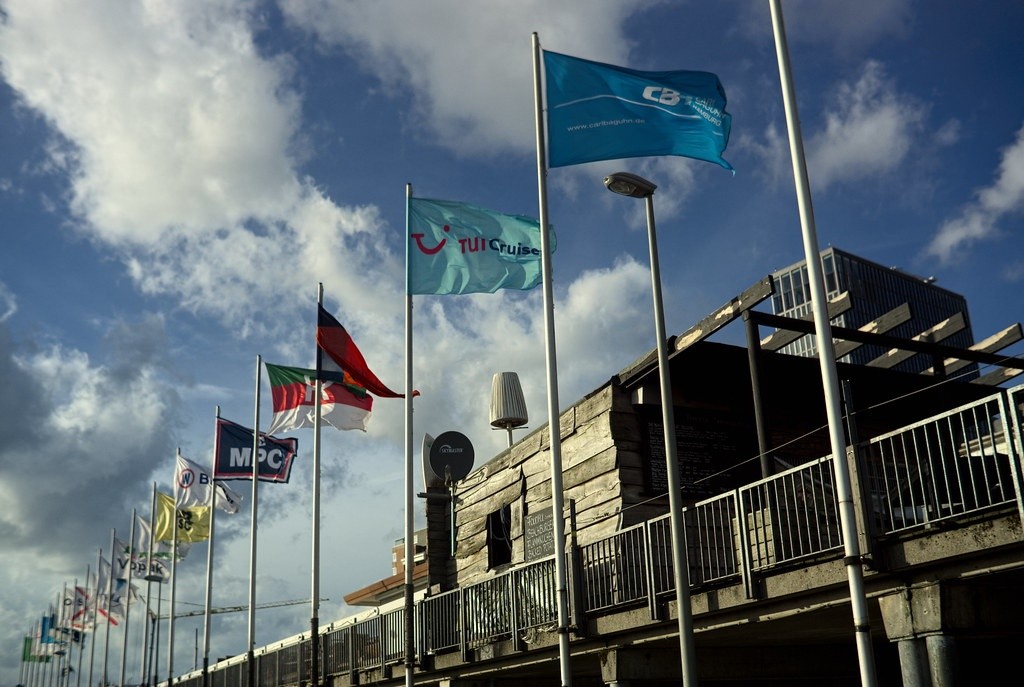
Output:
(732, 508), (788, 574)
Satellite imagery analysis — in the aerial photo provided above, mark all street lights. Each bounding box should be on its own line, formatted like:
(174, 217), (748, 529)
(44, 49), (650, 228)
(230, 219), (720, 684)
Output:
(604, 171), (699, 686)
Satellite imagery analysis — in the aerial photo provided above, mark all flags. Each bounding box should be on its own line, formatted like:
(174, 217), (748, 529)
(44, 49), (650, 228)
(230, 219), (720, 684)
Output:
(23, 515), (191, 664)
(154, 489), (210, 544)
(405, 196), (557, 295)
(173, 455), (244, 514)
(315, 300), (420, 398)
(539, 45), (736, 178)
(260, 362), (373, 436)
(213, 416), (299, 484)
